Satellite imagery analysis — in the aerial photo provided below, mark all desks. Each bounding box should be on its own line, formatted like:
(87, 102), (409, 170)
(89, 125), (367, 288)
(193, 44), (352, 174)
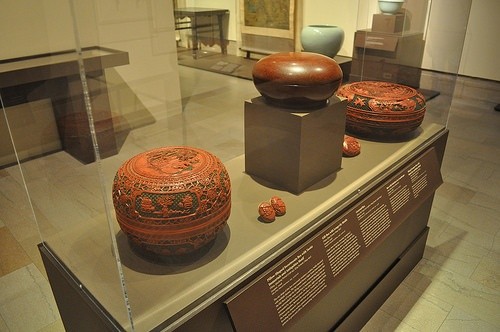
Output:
(173, 7), (229, 59)
(0, 46), (129, 165)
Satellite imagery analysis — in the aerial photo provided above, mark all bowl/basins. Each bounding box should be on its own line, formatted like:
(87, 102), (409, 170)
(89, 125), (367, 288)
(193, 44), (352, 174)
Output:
(378, 0), (404, 15)
